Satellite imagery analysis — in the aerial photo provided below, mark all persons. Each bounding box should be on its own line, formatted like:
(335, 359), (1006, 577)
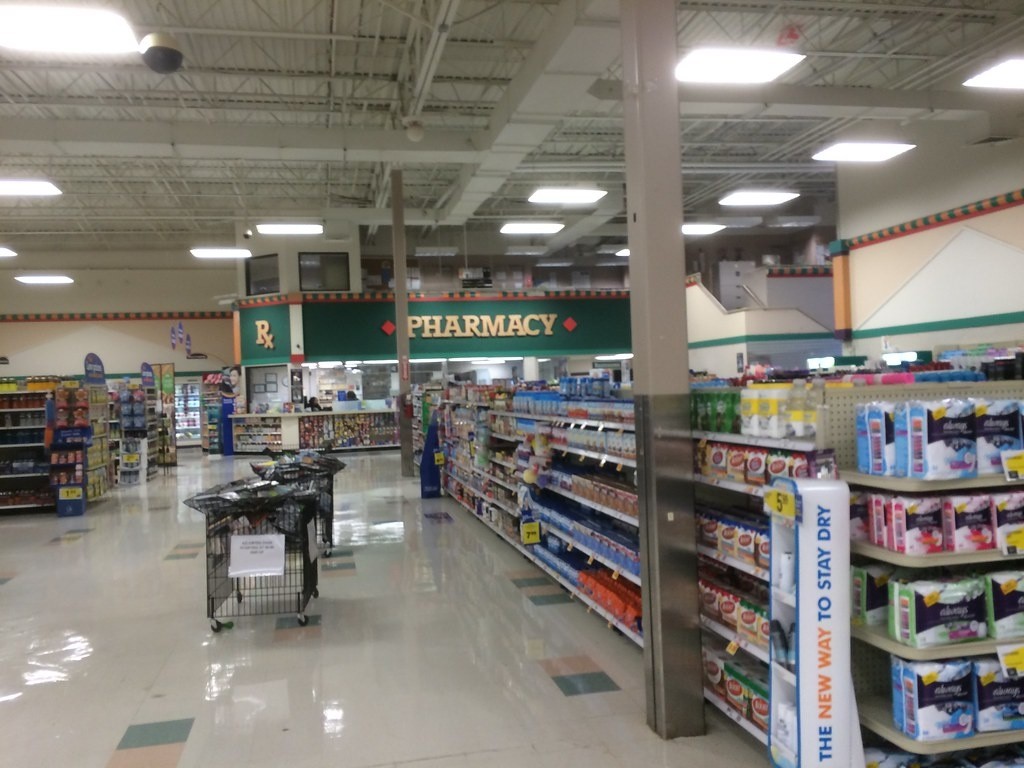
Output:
(346, 391), (359, 401)
(229, 365), (242, 395)
(308, 397), (322, 410)
(510, 366), (527, 387)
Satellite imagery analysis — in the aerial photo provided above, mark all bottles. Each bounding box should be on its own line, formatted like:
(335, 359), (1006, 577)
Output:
(0, 458), (49, 474)
(690, 381), (740, 434)
(0, 411), (46, 426)
(0, 394), (46, 409)
(0, 428), (44, 445)
(0, 490), (56, 506)
(0, 375), (61, 392)
(785, 378), (867, 441)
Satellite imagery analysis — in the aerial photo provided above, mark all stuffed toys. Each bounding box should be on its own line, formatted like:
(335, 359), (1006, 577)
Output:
(522, 433), (552, 489)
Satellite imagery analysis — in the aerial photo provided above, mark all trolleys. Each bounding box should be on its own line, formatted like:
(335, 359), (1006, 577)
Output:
(194, 444), (334, 632)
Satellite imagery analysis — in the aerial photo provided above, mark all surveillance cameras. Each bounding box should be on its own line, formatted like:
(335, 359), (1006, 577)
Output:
(243, 230), (252, 239)
(139, 35), (184, 73)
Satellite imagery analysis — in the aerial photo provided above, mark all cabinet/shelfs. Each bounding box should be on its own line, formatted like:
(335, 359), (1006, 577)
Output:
(0, 384), (160, 509)
(411, 389), (819, 747)
(840, 470), (1024, 755)
